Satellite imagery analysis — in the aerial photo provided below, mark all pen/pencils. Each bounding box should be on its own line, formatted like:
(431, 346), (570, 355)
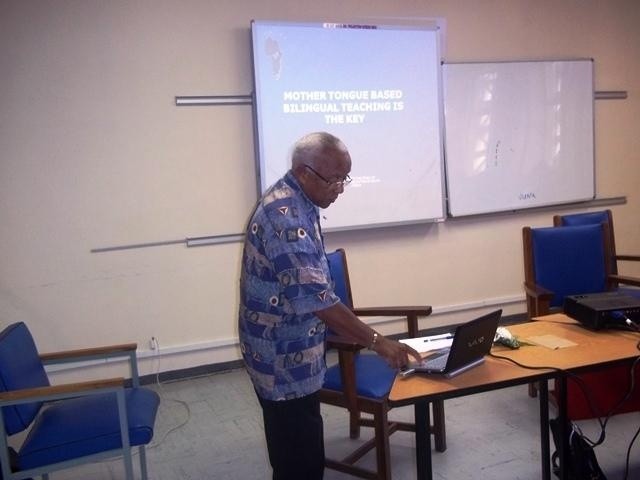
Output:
(424, 335), (455, 342)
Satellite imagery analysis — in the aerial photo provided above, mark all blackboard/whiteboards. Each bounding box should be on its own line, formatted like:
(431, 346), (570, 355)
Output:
(440, 58), (596, 218)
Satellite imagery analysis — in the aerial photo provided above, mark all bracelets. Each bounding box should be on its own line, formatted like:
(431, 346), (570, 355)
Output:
(370, 329), (379, 352)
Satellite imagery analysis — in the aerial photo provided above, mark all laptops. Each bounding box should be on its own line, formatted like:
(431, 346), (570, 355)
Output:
(406, 308), (504, 375)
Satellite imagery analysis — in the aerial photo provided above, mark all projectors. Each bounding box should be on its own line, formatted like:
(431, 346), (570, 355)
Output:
(564, 289), (640, 331)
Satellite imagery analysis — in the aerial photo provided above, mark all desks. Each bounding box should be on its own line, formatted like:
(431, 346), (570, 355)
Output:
(387, 314), (639, 480)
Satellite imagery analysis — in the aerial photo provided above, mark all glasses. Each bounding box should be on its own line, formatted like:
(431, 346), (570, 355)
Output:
(304, 164), (353, 188)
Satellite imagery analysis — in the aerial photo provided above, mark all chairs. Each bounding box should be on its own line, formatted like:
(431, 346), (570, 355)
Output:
(520, 207), (639, 397)
(319, 247), (446, 480)
(2, 322), (158, 479)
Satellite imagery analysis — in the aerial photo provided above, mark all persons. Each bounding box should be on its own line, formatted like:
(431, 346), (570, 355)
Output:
(239, 132), (425, 480)
